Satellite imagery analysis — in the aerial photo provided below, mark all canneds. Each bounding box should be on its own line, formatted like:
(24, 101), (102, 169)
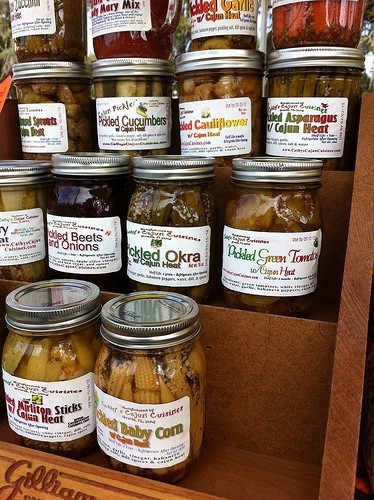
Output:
(0, 0), (367, 314)
(93, 291), (206, 484)
(1, 278), (106, 457)
